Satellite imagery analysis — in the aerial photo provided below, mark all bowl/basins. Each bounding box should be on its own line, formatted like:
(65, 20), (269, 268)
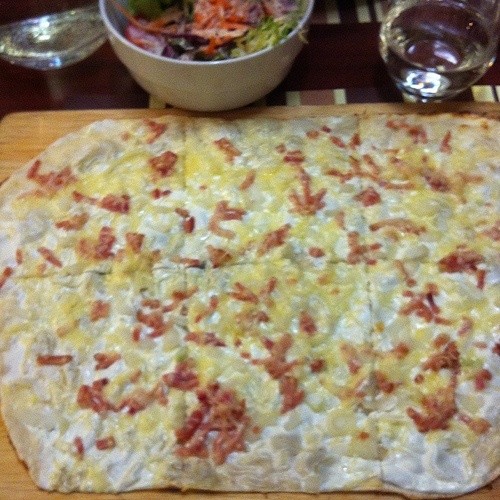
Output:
(99, 0), (315, 111)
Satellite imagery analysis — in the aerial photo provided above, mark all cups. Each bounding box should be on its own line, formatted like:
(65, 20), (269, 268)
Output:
(377, 0), (500, 99)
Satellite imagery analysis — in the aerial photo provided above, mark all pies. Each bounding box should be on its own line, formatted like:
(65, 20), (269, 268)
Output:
(0, 113), (500, 499)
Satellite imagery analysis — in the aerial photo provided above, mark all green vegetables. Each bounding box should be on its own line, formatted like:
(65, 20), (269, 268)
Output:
(231, 0), (308, 61)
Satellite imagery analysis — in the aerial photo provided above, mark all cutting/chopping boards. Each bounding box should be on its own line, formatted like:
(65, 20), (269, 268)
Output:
(0, 102), (500, 500)
